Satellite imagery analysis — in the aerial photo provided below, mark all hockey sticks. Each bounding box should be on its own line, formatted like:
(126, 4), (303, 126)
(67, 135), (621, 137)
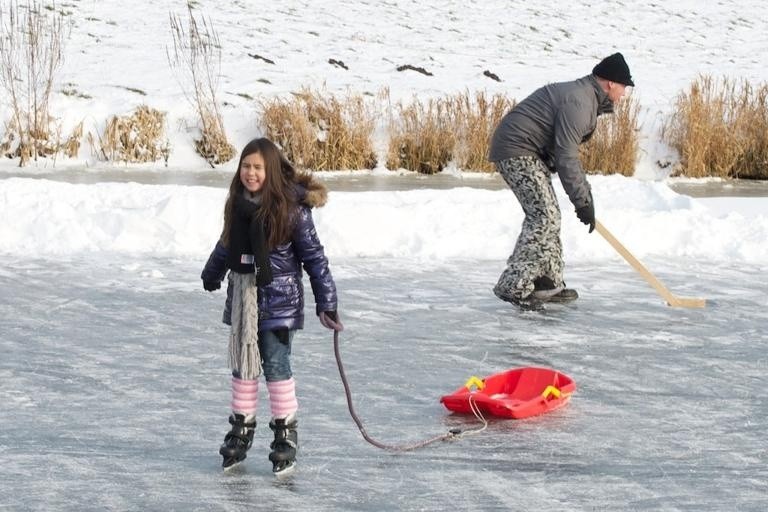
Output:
(595, 217), (706, 308)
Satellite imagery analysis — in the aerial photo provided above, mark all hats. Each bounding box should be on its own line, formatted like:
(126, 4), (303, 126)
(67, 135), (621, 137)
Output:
(592, 52), (636, 88)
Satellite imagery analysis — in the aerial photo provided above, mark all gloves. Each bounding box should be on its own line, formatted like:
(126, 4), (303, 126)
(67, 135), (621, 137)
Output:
(574, 202), (596, 234)
(203, 279), (222, 292)
(314, 304), (338, 324)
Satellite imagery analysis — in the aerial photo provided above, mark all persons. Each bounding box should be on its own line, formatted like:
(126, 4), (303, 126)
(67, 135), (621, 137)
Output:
(487, 52), (636, 310)
(200, 136), (341, 475)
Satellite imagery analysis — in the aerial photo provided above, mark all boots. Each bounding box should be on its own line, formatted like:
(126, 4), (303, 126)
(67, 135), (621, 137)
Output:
(219, 410), (257, 459)
(267, 414), (300, 462)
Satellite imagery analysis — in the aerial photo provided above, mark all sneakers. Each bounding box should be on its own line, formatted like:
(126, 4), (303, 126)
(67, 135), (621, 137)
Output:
(549, 288), (580, 303)
(491, 288), (546, 311)
